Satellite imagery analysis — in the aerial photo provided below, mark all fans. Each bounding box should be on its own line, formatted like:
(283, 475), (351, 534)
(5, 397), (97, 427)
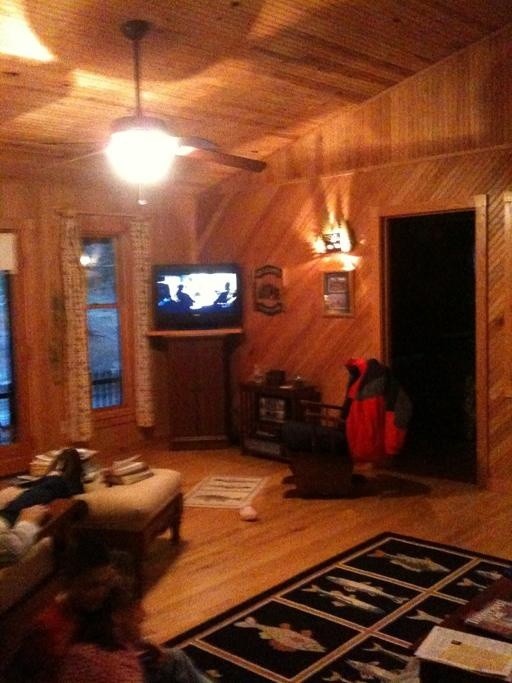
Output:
(32, 17), (269, 181)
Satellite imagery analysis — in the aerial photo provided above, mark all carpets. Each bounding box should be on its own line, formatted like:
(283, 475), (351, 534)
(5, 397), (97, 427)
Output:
(143, 533), (511, 683)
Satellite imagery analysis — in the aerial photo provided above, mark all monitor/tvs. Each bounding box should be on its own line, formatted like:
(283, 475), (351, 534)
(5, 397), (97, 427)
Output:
(151, 263), (242, 330)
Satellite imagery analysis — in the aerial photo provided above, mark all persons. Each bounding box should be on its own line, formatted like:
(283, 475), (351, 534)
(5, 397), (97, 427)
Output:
(1, 449), (82, 560)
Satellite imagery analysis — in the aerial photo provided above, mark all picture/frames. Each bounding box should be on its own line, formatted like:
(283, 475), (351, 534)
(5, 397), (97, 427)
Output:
(319, 268), (354, 317)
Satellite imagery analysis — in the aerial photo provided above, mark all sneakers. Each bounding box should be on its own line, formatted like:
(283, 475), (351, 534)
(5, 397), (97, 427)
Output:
(61, 450), (81, 478)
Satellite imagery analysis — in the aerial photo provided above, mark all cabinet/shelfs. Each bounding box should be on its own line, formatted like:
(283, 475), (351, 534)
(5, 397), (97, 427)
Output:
(237, 380), (317, 462)
(166, 339), (229, 451)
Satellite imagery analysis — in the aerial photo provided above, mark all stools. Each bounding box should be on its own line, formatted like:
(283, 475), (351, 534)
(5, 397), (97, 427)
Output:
(3, 453), (183, 576)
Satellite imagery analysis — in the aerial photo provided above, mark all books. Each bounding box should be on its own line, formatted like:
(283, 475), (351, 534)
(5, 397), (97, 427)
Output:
(28, 448), (153, 484)
